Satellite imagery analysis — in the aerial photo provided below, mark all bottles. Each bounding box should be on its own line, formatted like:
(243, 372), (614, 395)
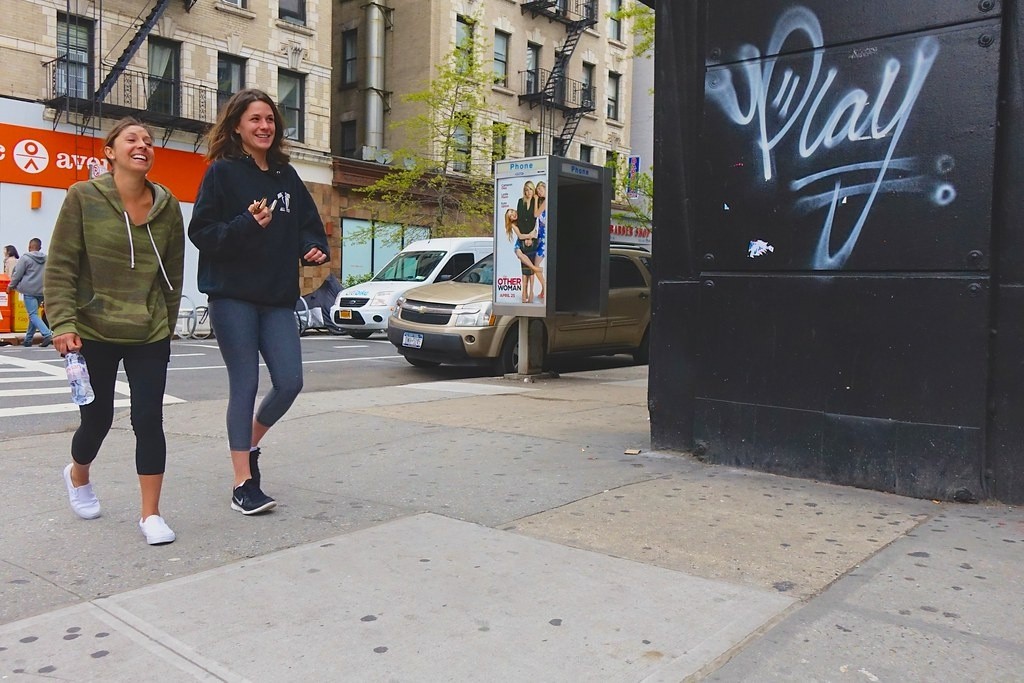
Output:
(65, 350), (95, 405)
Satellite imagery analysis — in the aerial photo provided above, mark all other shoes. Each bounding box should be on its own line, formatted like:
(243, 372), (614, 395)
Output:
(22, 343), (32, 347)
(39, 337), (53, 347)
(538, 293), (545, 298)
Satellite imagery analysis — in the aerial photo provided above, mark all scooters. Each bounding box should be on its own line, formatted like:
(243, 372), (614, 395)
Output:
(294, 273), (348, 335)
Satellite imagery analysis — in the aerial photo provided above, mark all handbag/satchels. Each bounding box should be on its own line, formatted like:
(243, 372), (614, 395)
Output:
(521, 238), (538, 253)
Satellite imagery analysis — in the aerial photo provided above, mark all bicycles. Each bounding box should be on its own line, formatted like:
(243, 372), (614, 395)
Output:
(187, 305), (213, 340)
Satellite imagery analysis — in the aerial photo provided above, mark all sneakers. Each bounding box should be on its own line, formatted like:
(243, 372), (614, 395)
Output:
(231, 479), (277, 515)
(63, 463), (101, 519)
(139, 515), (176, 545)
(249, 448), (262, 489)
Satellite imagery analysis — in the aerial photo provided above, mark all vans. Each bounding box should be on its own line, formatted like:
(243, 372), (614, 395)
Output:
(329, 236), (494, 338)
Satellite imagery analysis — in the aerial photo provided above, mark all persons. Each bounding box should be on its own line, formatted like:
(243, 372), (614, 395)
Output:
(43, 116), (185, 545)
(4, 238), (53, 347)
(505, 181), (546, 304)
(187, 89), (330, 515)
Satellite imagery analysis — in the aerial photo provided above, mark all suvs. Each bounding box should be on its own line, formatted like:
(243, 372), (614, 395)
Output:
(385, 240), (651, 378)
